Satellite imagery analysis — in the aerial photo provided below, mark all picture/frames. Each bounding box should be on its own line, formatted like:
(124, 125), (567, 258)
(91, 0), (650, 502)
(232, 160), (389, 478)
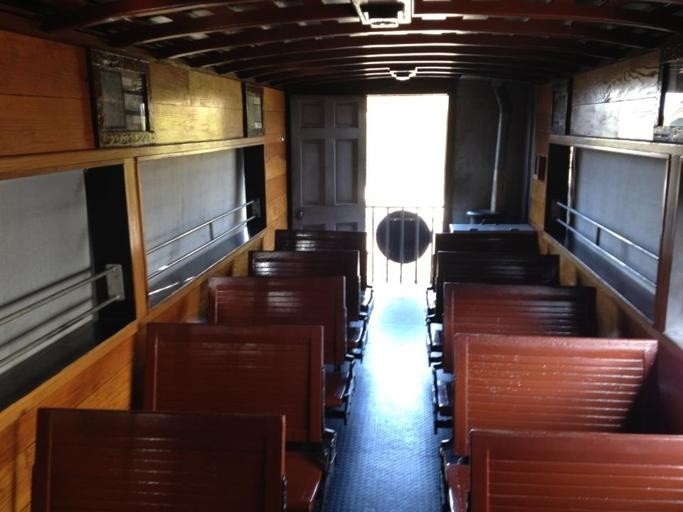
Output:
(87, 48), (157, 148)
(653, 46), (683, 143)
(549, 78), (573, 135)
(242, 82), (266, 137)
